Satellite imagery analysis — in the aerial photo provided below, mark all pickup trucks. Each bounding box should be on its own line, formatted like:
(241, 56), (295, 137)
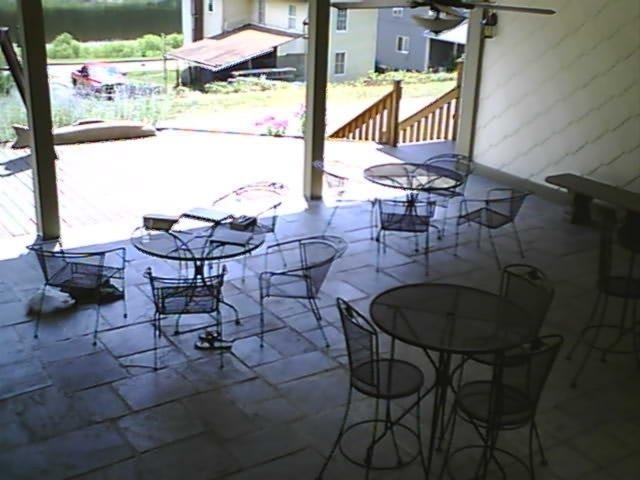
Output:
(70, 62), (162, 99)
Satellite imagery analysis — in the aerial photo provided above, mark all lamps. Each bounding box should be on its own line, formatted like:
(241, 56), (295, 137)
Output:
(481, 9), (497, 38)
(409, 14), (466, 35)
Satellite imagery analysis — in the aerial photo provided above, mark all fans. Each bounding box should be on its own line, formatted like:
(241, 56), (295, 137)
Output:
(330, 0), (556, 18)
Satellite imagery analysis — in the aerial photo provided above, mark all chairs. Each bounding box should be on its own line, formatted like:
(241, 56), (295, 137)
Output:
(312, 159), (379, 241)
(454, 187), (535, 271)
(408, 153), (479, 237)
(450, 262), (555, 383)
(375, 199), (436, 278)
(440, 333), (566, 479)
(259, 234), (350, 349)
(565, 213), (637, 389)
(25, 239), (128, 347)
(143, 263), (227, 372)
(211, 180), (290, 285)
(318, 297), (429, 478)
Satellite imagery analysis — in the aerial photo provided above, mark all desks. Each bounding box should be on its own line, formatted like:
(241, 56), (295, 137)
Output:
(363, 163), (464, 242)
(369, 283), (535, 452)
(131, 217), (265, 335)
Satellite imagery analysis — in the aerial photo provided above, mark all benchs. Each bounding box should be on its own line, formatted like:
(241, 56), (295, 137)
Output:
(545, 173), (639, 226)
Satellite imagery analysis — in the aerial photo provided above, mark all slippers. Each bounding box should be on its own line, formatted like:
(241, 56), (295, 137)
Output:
(194, 337), (232, 349)
(199, 329), (233, 342)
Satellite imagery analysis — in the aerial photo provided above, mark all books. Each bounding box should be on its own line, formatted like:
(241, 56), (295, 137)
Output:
(209, 230), (252, 247)
